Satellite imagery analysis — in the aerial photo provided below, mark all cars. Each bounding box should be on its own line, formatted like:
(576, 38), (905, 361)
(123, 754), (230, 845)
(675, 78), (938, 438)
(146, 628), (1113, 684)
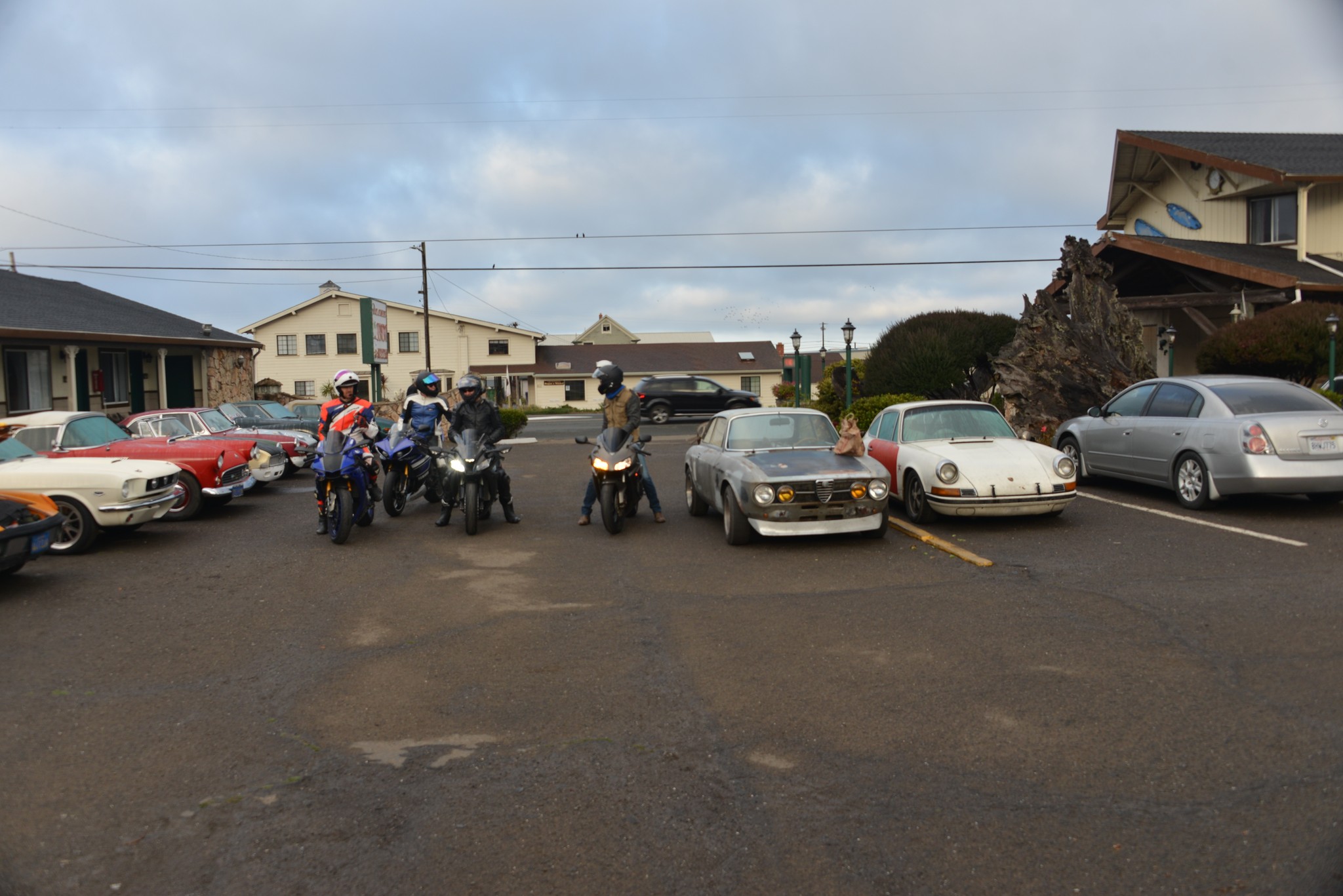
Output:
(0, 488), (64, 584)
(1053, 374), (1342, 510)
(0, 437), (182, 555)
(0, 409), (259, 522)
(1319, 375), (1343, 393)
(859, 400), (1077, 527)
(685, 408), (895, 542)
(117, 398), (399, 491)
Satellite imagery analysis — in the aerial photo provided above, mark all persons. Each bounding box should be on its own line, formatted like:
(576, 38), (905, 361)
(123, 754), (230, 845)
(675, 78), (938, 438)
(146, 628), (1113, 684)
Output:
(380, 371), (458, 507)
(406, 378), (418, 395)
(578, 366), (666, 526)
(315, 370), (384, 534)
(435, 374), (520, 526)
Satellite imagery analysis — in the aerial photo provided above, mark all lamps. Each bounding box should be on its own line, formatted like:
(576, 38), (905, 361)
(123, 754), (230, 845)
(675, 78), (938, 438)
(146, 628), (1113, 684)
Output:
(234, 355), (245, 370)
(202, 324), (213, 333)
(143, 352), (153, 363)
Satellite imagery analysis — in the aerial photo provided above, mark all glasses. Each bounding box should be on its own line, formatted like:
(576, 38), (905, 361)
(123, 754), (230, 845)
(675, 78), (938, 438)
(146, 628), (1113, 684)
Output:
(460, 387), (475, 392)
(427, 382), (438, 387)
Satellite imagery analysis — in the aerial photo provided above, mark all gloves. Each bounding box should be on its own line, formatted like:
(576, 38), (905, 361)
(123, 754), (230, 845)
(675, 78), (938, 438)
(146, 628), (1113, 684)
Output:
(354, 415), (369, 432)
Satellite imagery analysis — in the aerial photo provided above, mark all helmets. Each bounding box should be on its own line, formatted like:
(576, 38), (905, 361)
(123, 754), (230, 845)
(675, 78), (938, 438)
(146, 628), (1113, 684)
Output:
(592, 364), (623, 395)
(333, 369), (360, 395)
(457, 375), (486, 404)
(416, 371), (441, 397)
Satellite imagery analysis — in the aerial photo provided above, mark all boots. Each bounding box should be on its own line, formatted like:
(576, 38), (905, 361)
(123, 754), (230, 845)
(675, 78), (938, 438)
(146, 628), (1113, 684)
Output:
(435, 496), (454, 526)
(504, 506), (520, 523)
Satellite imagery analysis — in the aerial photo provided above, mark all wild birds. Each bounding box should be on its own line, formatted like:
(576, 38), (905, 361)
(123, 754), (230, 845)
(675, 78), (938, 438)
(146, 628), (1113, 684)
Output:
(576, 233), (578, 237)
(492, 264), (495, 269)
(583, 233), (585, 237)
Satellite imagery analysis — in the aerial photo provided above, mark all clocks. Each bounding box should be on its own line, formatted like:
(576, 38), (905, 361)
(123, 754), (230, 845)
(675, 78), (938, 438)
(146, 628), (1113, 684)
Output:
(1205, 166), (1224, 194)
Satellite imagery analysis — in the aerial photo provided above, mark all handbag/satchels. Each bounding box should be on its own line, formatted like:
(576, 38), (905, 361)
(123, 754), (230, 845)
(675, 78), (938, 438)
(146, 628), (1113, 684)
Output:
(834, 413), (865, 457)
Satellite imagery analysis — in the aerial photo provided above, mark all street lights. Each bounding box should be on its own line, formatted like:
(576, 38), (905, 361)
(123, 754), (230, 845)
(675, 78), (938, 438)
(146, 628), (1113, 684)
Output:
(1165, 323), (1177, 378)
(818, 347), (827, 380)
(1323, 313), (1339, 395)
(789, 328), (802, 407)
(840, 317), (856, 410)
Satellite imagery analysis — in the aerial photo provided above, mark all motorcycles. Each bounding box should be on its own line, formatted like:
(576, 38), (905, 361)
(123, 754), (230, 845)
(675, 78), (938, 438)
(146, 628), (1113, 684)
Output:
(294, 429), (379, 544)
(575, 427), (653, 534)
(374, 423), (447, 514)
(430, 427), (514, 534)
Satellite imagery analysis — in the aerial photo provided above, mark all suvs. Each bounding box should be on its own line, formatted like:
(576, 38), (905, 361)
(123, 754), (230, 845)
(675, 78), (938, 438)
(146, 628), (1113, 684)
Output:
(633, 374), (763, 425)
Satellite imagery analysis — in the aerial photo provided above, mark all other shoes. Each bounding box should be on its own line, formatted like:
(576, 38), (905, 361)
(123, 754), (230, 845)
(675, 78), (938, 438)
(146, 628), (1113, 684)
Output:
(432, 468), (444, 498)
(655, 512), (666, 523)
(578, 515), (590, 524)
(367, 484), (382, 502)
(316, 517), (328, 534)
